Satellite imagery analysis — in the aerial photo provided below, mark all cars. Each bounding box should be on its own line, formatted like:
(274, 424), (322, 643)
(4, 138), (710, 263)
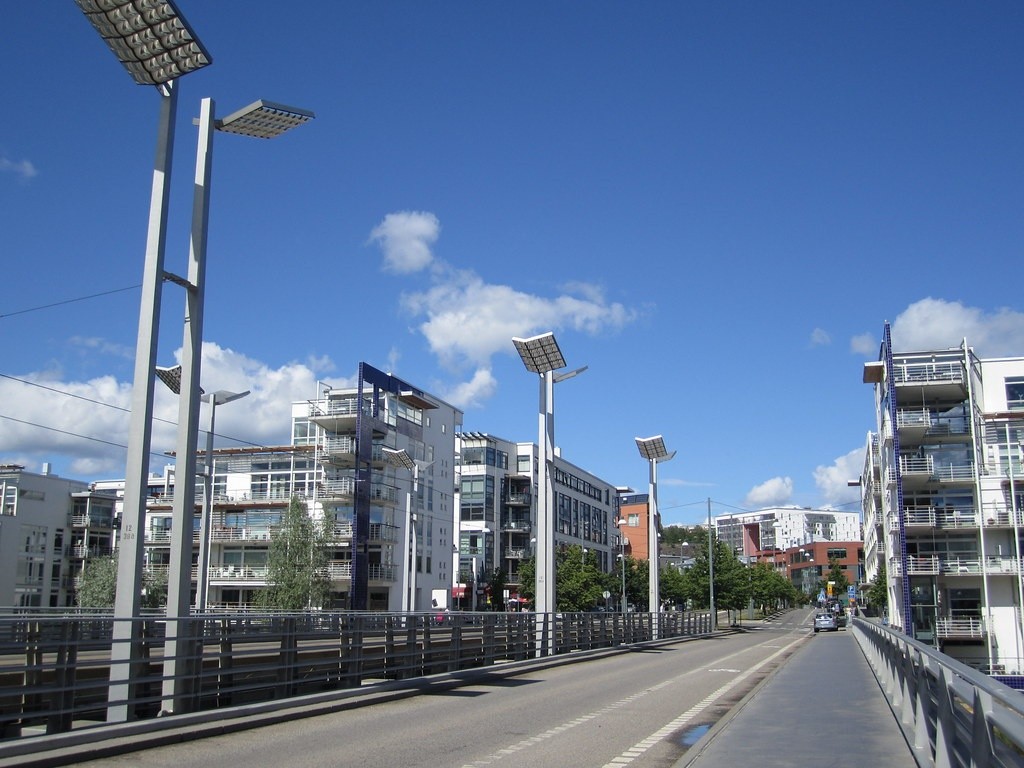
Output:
(666, 603), (682, 610)
(827, 599), (846, 623)
(814, 613), (838, 632)
(556, 606), (615, 620)
(628, 603), (636, 612)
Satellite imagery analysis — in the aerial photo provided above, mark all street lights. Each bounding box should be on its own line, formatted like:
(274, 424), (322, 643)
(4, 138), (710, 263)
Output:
(634, 434), (677, 641)
(382, 448), (437, 627)
(512, 332), (590, 658)
(154, 364), (250, 615)
(74, 0), (314, 729)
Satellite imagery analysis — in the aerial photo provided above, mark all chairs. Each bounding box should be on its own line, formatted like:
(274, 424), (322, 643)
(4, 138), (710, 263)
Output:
(957, 557), (969, 574)
(1000, 561), (1011, 572)
